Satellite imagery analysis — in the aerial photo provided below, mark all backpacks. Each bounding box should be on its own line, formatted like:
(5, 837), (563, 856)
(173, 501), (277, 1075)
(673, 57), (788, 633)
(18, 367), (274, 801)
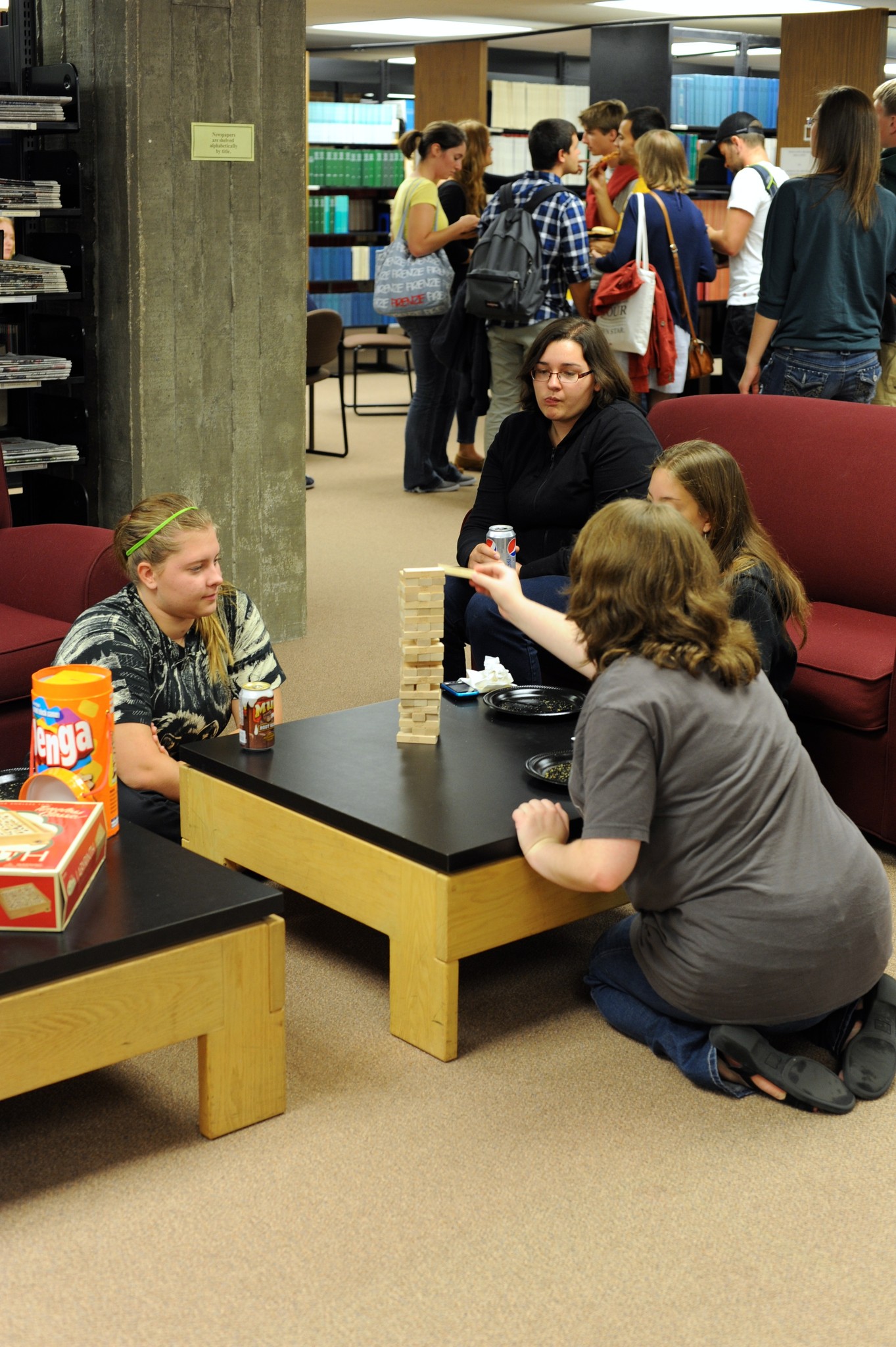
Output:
(464, 182), (577, 322)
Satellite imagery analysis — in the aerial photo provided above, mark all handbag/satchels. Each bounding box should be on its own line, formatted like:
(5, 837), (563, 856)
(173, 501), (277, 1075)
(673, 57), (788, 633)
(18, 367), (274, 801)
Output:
(688, 339), (715, 379)
(596, 270), (657, 355)
(372, 182), (455, 318)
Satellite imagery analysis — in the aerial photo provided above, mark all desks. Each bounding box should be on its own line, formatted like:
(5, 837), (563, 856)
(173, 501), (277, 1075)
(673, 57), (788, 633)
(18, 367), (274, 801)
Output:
(1, 817), (289, 1140)
(180, 694), (631, 1063)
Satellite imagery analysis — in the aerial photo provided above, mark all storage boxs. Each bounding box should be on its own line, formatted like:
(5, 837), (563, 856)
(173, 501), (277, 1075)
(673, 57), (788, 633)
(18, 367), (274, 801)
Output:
(1, 799), (110, 932)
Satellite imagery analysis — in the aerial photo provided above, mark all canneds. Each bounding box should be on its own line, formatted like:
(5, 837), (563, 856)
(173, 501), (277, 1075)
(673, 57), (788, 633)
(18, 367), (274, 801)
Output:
(239, 681), (275, 751)
(486, 525), (516, 570)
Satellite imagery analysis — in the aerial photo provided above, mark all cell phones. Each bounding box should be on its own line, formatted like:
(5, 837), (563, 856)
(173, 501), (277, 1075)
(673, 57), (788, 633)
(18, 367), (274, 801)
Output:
(441, 681), (479, 697)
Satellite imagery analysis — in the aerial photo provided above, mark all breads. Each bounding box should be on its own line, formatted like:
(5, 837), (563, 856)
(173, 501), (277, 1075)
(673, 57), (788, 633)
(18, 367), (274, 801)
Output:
(589, 241), (615, 254)
(591, 226), (614, 235)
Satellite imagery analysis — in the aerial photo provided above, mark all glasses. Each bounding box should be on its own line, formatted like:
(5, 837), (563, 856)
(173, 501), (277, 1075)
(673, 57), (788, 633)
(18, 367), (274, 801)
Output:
(530, 367), (593, 384)
(807, 117), (819, 126)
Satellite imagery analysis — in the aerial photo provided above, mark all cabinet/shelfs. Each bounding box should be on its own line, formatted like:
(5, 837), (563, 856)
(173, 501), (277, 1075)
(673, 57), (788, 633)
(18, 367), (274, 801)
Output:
(489, 127), (590, 200)
(669, 122), (779, 396)
(308, 141), (404, 378)
(0, 119), (91, 525)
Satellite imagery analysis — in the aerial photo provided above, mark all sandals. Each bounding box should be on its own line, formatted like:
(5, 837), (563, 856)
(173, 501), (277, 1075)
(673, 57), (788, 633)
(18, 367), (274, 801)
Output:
(710, 1021), (857, 1117)
(841, 979), (896, 1100)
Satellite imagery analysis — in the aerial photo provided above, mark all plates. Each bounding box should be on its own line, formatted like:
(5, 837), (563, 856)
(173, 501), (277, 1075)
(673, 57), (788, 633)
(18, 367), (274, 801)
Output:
(482, 685), (587, 719)
(588, 227), (619, 238)
(524, 749), (572, 789)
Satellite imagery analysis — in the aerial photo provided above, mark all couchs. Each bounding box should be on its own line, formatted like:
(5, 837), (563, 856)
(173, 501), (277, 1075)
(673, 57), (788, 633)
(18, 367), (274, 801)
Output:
(0, 443), (132, 772)
(645, 393), (896, 850)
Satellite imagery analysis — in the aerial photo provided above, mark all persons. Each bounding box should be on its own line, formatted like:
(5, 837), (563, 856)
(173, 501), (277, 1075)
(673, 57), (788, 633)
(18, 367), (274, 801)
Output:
(477, 118), (592, 457)
(468, 498), (896, 1116)
(579, 99), (668, 231)
(738, 86), (896, 404)
(436, 120), (591, 473)
(0, 216), (15, 260)
(647, 439), (808, 704)
(591, 130), (717, 410)
(389, 121), (481, 492)
(455, 317), (664, 686)
(870, 78), (896, 407)
(705, 111), (789, 394)
(50, 494), (286, 845)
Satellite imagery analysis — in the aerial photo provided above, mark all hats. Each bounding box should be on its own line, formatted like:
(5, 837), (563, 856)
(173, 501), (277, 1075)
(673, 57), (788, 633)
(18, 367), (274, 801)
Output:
(705, 111), (764, 159)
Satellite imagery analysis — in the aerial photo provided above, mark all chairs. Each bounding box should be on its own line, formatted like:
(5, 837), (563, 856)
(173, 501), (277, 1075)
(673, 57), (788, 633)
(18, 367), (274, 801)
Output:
(304, 308), (349, 458)
(338, 327), (414, 416)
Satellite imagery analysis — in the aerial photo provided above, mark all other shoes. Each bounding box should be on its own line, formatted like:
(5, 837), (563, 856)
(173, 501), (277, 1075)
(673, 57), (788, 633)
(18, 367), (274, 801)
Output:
(442, 464), (477, 486)
(402, 479), (459, 492)
(456, 454), (484, 470)
(305, 476), (314, 489)
